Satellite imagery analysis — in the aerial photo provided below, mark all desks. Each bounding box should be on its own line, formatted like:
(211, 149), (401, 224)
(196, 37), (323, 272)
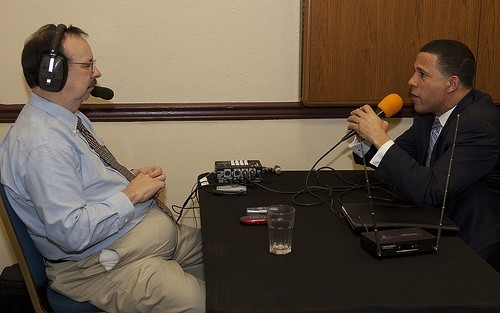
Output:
(197, 170), (500, 313)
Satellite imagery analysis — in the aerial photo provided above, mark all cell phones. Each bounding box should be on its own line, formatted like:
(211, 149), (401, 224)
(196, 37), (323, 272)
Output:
(216, 185), (248, 194)
(246, 205), (279, 216)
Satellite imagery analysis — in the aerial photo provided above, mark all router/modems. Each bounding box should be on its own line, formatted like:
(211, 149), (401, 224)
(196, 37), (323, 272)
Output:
(358, 114), (461, 259)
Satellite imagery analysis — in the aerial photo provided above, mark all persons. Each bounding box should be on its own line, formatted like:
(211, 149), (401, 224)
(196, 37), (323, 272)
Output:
(0, 24), (206, 313)
(348, 40), (500, 273)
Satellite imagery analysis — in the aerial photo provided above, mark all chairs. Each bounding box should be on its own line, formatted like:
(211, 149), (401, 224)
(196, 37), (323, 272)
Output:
(0, 183), (108, 313)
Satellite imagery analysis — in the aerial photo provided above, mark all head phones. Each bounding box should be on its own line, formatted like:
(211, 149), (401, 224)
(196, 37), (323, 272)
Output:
(37, 24), (67, 92)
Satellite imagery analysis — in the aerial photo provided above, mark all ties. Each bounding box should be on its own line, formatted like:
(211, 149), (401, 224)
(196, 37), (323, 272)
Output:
(77, 116), (182, 231)
(425, 117), (440, 168)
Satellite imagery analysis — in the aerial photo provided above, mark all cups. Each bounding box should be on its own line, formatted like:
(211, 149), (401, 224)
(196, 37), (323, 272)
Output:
(266, 205), (295, 255)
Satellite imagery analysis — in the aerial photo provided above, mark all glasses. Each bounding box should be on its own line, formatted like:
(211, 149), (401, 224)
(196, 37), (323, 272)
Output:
(69, 60), (97, 73)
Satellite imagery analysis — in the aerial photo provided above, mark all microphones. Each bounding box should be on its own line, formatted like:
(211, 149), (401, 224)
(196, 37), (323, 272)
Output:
(343, 94), (403, 140)
(91, 86), (113, 100)
